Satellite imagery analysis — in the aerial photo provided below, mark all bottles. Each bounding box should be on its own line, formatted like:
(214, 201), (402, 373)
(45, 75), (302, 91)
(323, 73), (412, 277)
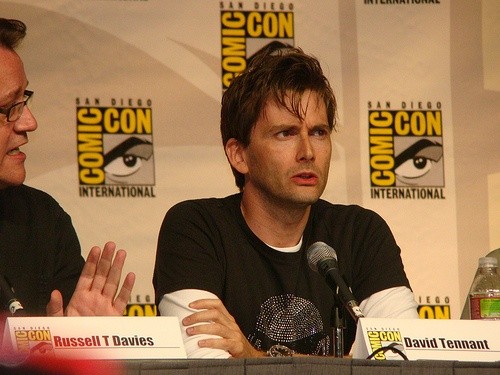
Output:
(469, 257), (500, 320)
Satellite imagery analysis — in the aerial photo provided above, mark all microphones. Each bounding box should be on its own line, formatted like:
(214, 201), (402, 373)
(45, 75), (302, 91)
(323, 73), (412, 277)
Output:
(307, 242), (364, 322)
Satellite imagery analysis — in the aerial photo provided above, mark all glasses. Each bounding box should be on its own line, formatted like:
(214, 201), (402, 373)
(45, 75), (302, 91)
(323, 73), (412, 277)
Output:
(0, 89), (35, 123)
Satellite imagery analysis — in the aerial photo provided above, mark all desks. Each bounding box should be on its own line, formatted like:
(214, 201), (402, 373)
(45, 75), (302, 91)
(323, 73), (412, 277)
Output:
(0, 353), (500, 375)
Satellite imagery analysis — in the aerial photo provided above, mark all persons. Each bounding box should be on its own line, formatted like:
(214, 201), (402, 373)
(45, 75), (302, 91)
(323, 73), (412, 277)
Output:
(0, 18), (135, 317)
(153, 48), (418, 358)
